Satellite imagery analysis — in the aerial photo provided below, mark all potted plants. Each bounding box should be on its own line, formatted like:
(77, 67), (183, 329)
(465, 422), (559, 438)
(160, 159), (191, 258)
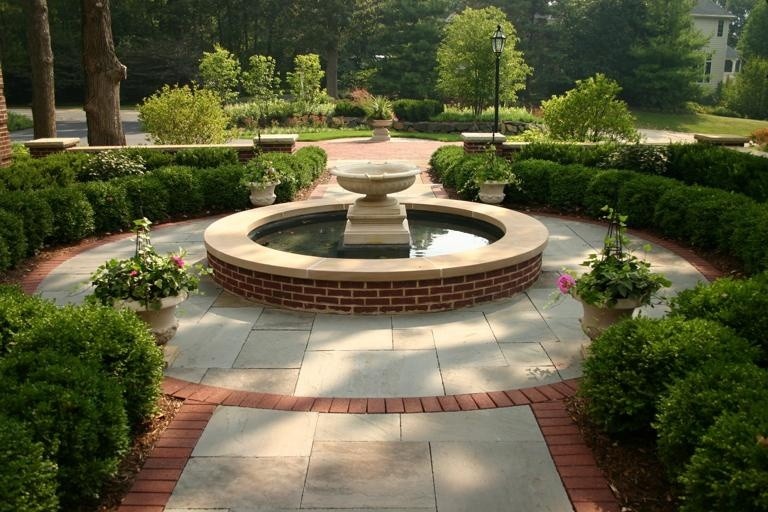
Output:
(457, 144), (526, 205)
(360, 95), (399, 142)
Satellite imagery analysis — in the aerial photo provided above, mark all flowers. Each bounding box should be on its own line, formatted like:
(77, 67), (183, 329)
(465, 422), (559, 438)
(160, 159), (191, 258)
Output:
(64, 216), (218, 318)
(543, 204), (677, 316)
(237, 154), (284, 192)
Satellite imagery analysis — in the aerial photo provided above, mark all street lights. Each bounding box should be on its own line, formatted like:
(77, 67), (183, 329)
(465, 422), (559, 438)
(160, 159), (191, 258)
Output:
(489, 22), (506, 135)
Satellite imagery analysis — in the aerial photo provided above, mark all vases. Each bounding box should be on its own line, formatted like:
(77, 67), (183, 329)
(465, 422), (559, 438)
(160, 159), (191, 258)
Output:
(580, 297), (641, 342)
(245, 180), (282, 207)
(114, 289), (189, 371)
